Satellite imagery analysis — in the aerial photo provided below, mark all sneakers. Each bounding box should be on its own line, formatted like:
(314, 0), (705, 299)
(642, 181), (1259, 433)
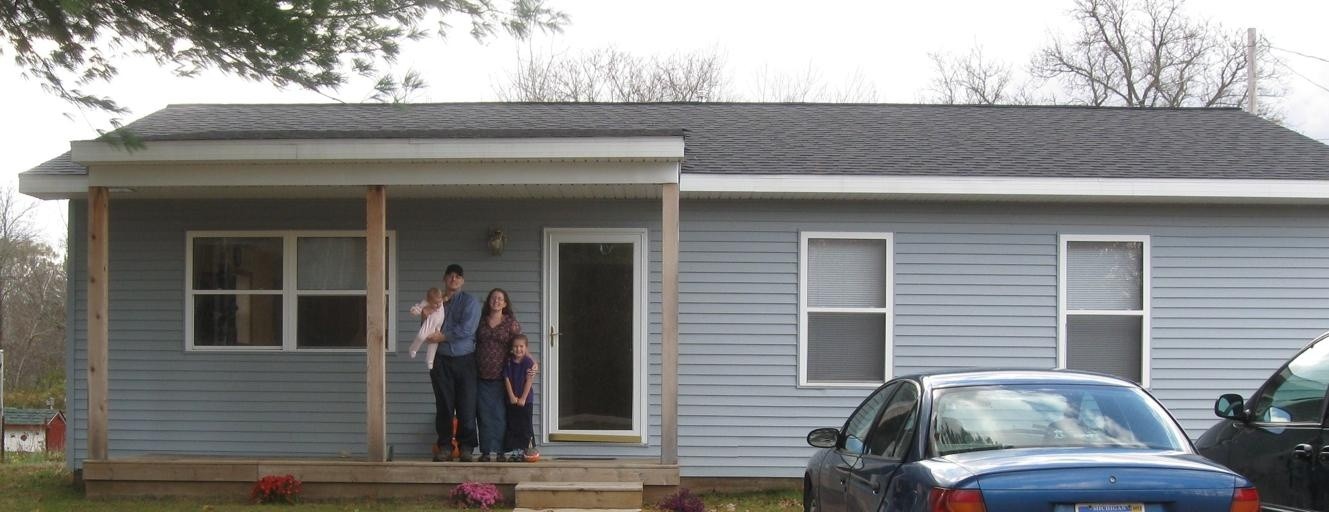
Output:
(496, 453), (506, 462)
(479, 452), (490, 462)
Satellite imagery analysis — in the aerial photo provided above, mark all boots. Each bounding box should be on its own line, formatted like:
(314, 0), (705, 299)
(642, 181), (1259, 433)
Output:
(433, 446), (452, 461)
(459, 448), (474, 462)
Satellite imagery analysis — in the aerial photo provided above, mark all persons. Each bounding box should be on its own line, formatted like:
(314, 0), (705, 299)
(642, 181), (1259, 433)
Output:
(409, 264), (538, 461)
